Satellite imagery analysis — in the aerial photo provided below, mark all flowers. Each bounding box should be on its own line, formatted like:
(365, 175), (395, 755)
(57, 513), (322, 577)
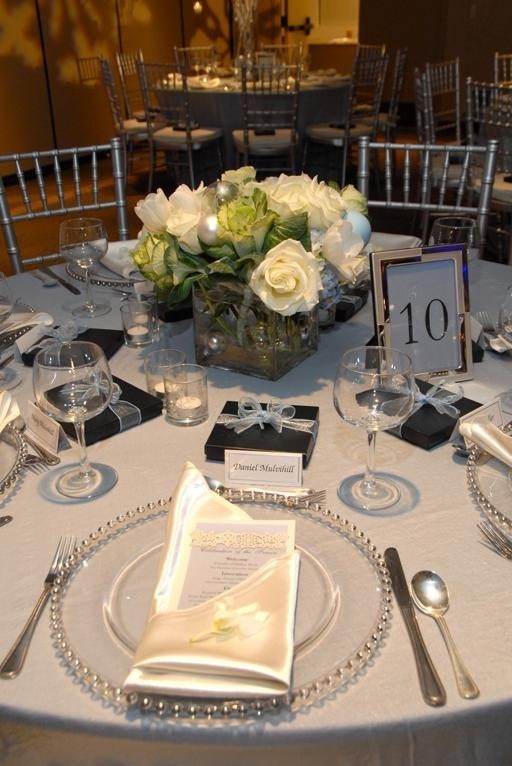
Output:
(136, 166), (372, 319)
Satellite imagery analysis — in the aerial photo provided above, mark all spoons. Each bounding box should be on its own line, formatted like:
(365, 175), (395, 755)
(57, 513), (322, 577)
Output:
(64, 261), (149, 289)
(410, 571), (481, 701)
(29, 268), (59, 292)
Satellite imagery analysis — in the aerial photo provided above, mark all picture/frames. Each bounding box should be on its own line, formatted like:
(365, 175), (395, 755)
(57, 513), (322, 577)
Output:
(369, 244), (473, 388)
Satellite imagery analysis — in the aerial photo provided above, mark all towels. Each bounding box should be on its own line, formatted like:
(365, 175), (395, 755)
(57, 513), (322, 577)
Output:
(0, 303), (56, 341)
(121, 462), (300, 699)
(0, 388), (22, 433)
(365, 232), (420, 262)
(459, 415), (512, 469)
(490, 332), (511, 355)
(101, 239), (147, 280)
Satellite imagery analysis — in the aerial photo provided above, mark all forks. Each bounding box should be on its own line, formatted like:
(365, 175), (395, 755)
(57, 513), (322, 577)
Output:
(475, 515), (512, 562)
(12, 416), (60, 466)
(474, 309), (512, 360)
(1, 534), (81, 683)
(21, 454), (49, 476)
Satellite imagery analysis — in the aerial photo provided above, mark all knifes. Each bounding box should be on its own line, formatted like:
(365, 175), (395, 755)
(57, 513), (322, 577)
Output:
(46, 492), (397, 721)
(40, 265), (81, 296)
(378, 548), (447, 712)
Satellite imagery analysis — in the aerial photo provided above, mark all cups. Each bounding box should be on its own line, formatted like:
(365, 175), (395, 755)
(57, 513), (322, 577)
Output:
(117, 301), (155, 349)
(142, 347), (188, 401)
(329, 345), (419, 516)
(31, 343), (115, 501)
(501, 277), (512, 335)
(57, 217), (114, 320)
(426, 217), (483, 277)
(163, 362), (209, 428)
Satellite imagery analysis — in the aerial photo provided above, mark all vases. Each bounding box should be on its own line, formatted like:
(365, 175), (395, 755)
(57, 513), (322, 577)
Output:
(191, 289), (320, 384)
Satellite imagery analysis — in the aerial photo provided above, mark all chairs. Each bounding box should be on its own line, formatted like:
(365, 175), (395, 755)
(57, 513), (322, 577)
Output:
(233, 62), (300, 180)
(302, 47), (389, 187)
(351, 46), (408, 187)
(0, 136), (125, 273)
(116, 48), (160, 176)
(260, 41), (303, 65)
(465, 77), (512, 265)
(493, 52), (512, 82)
(425, 57), (476, 160)
(97, 53), (165, 191)
(134, 60), (224, 194)
(358, 133), (498, 258)
(413, 73), (484, 242)
(172, 43), (219, 77)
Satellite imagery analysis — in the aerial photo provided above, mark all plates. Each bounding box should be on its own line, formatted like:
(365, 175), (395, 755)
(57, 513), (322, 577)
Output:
(0, 405), (30, 494)
(465, 418), (512, 541)
(0, 297), (36, 345)
